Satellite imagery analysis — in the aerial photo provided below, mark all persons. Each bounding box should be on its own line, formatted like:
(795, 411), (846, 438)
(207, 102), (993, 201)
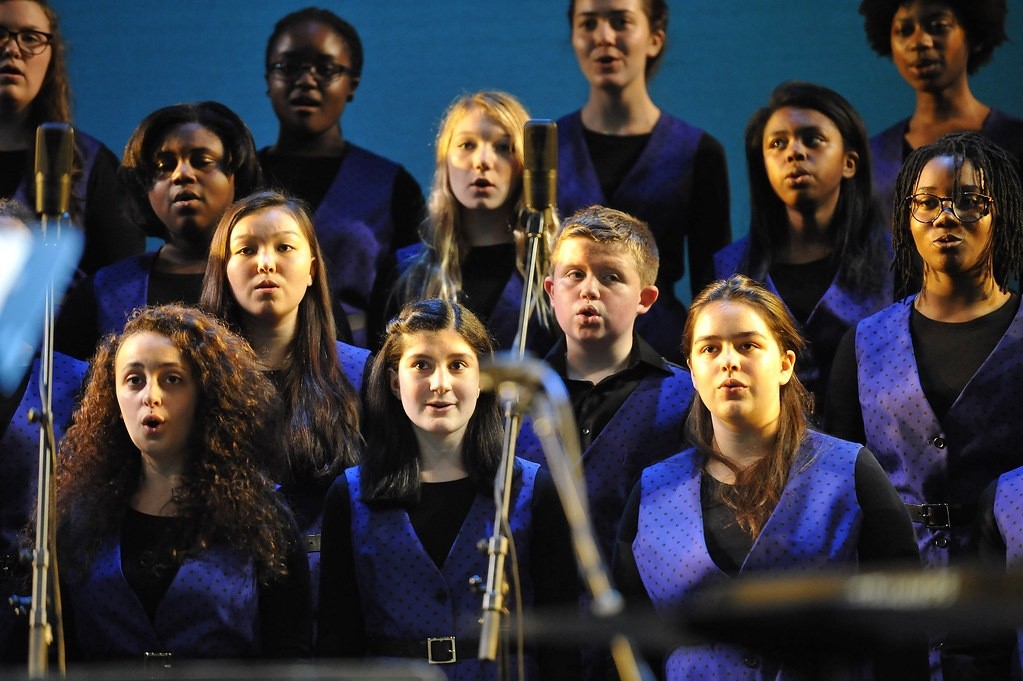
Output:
(611, 275), (931, 681)
(816, 131), (1023, 681)
(704, 82), (895, 393)
(859, 0), (1023, 224)
(0, 0), (730, 680)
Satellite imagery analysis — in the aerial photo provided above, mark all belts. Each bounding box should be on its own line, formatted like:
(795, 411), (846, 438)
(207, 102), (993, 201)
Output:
(97, 651), (241, 675)
(300, 534), (322, 552)
(902, 502), (988, 529)
(370, 636), (479, 663)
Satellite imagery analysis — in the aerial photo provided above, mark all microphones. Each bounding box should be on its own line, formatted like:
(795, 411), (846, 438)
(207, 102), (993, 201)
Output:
(35, 121), (75, 258)
(523, 120), (560, 278)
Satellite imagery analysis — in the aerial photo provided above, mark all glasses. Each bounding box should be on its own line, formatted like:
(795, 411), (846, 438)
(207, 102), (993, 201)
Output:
(0, 29), (54, 55)
(906, 192), (998, 224)
(267, 59), (356, 82)
